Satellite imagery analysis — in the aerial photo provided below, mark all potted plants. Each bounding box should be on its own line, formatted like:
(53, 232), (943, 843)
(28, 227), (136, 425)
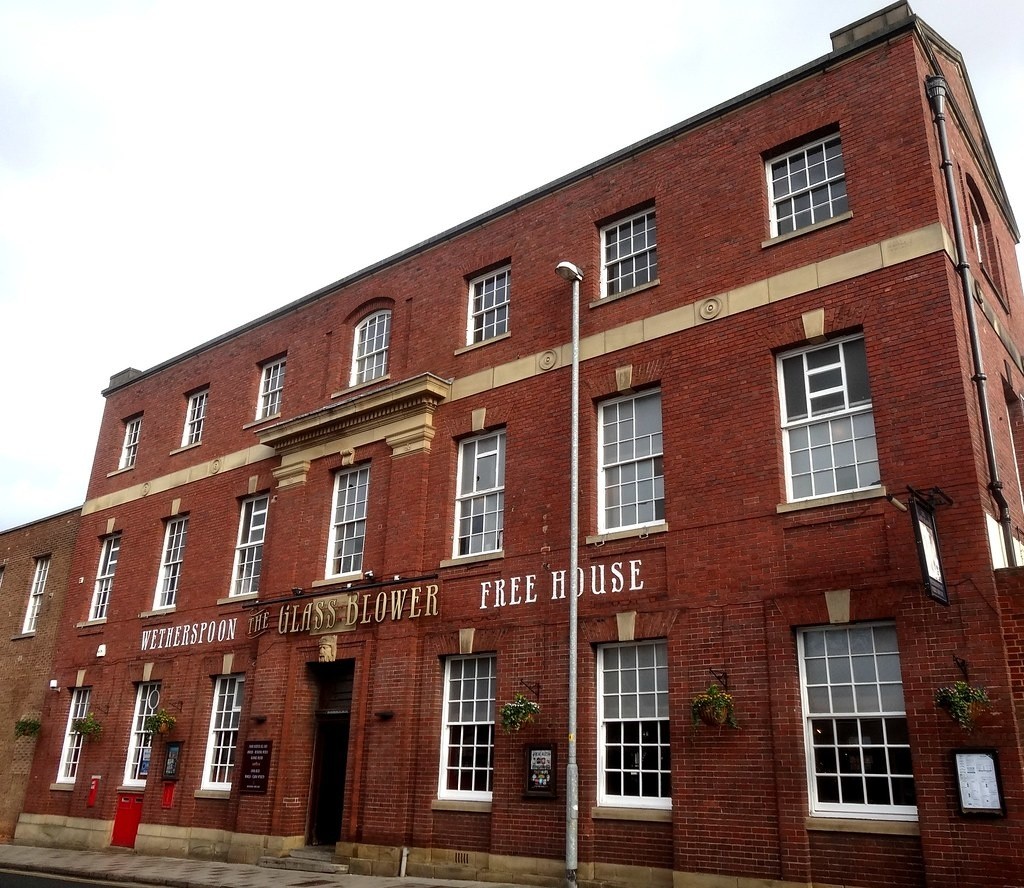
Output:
(14, 718), (41, 739)
(71, 712), (104, 742)
(145, 709), (177, 732)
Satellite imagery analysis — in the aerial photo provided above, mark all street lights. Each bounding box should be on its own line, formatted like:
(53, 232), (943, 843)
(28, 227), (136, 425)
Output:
(556, 261), (585, 888)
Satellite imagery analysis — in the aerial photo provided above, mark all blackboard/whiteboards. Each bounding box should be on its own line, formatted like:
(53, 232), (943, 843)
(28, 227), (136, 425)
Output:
(237, 739), (274, 794)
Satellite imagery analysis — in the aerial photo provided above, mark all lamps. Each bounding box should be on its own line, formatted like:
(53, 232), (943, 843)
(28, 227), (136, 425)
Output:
(251, 716), (266, 721)
(291, 587), (303, 596)
(374, 711), (394, 719)
(364, 570), (374, 578)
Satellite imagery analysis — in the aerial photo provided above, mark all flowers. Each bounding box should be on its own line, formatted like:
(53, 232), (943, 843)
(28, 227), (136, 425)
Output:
(499, 694), (541, 734)
(689, 683), (741, 731)
(934, 681), (993, 736)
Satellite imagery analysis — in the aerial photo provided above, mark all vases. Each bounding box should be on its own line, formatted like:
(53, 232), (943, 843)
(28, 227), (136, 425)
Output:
(518, 721), (528, 728)
(695, 706), (728, 726)
(947, 702), (984, 721)
